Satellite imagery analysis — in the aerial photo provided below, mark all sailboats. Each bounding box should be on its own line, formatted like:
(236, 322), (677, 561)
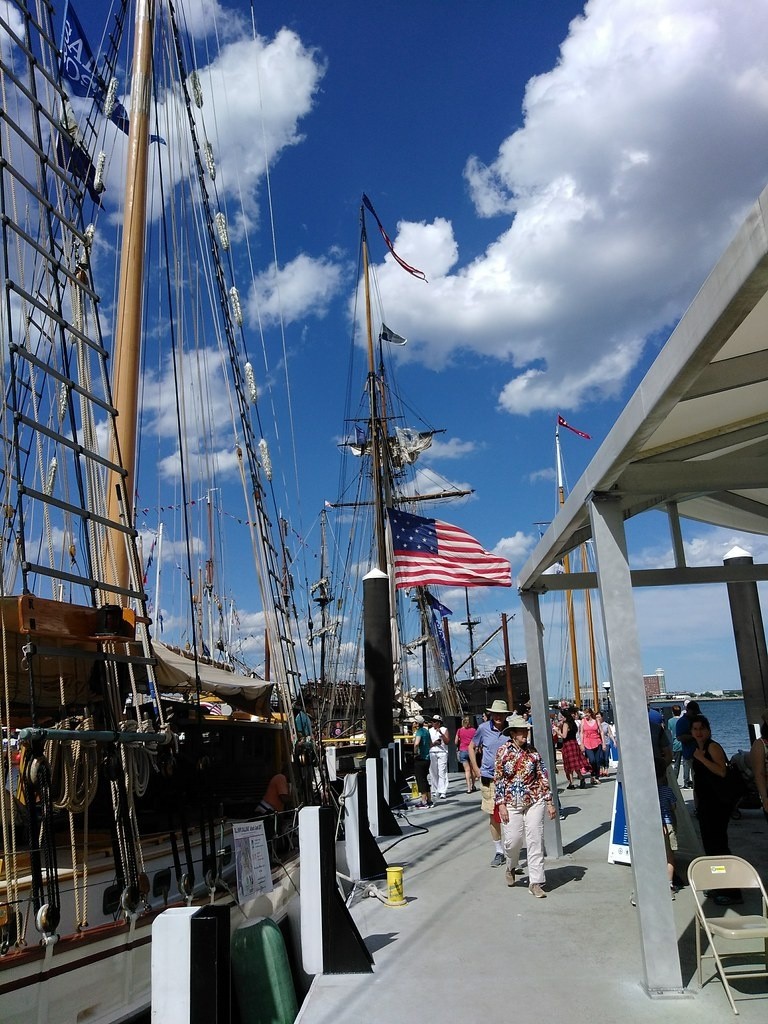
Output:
(0, 0), (611, 1024)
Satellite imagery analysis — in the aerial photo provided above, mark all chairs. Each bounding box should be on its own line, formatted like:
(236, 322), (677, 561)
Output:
(688, 855), (768, 1014)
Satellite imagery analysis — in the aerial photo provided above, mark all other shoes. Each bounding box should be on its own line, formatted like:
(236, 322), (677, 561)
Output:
(684, 779), (693, 789)
(713, 896), (744, 906)
(432, 792), (439, 796)
(440, 793), (446, 798)
(568, 765), (609, 789)
(702, 890), (719, 897)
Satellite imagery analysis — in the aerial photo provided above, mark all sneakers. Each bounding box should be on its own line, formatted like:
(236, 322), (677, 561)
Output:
(415, 801), (429, 809)
(427, 802), (434, 807)
(490, 852), (506, 868)
(515, 864), (525, 874)
(528, 882), (547, 898)
(505, 867), (515, 886)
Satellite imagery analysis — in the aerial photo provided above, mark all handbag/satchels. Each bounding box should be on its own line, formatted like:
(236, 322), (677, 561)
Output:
(704, 738), (746, 812)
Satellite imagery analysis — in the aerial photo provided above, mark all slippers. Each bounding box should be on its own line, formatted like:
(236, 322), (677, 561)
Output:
(466, 789), (471, 794)
(472, 786), (476, 791)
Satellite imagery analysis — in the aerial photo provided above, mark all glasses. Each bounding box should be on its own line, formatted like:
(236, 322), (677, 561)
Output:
(512, 730), (528, 734)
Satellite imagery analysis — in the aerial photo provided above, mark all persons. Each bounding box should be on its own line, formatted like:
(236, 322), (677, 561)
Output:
(413, 700), (742, 905)
(750, 720), (768, 818)
(249, 700), (346, 867)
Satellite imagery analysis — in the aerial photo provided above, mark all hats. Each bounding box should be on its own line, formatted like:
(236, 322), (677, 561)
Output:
(524, 699), (532, 708)
(409, 715), (425, 724)
(687, 701), (702, 714)
(503, 715), (533, 736)
(486, 700), (512, 713)
(431, 714), (443, 724)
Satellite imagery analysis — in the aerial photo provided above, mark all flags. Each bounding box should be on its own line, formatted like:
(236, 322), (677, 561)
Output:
(56, 0), (167, 144)
(382, 323), (408, 346)
(199, 702), (222, 716)
(385, 506), (512, 587)
(55, 93), (107, 212)
(557, 414), (590, 440)
